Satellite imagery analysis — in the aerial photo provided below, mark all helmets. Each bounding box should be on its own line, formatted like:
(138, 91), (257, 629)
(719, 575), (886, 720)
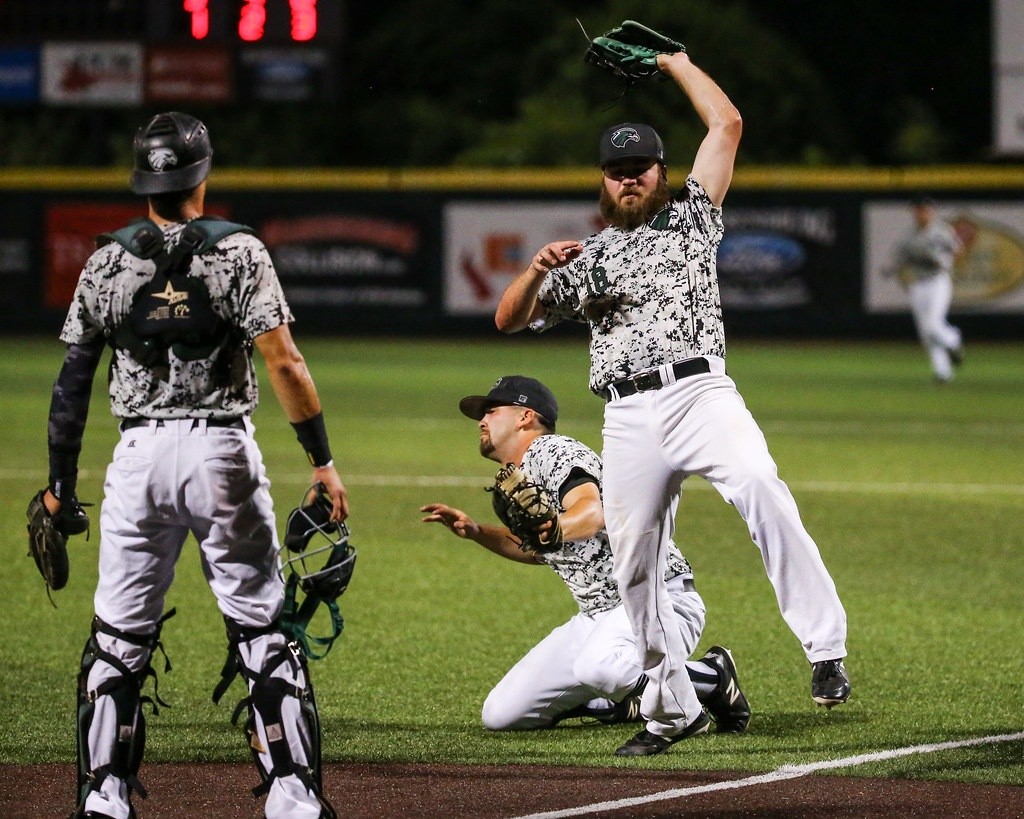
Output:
(128, 110), (212, 195)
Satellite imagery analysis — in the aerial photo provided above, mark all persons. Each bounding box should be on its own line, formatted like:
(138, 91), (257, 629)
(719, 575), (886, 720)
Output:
(24, 112), (350, 819)
(419, 374), (752, 738)
(882, 198), (965, 384)
(494, 52), (851, 758)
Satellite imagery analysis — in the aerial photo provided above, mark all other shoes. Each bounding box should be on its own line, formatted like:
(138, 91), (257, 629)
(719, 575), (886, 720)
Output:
(951, 339), (965, 363)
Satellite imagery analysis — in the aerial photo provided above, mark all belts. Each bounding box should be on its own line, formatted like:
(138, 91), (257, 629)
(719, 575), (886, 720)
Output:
(121, 416), (245, 429)
(606, 357), (728, 402)
(684, 578), (696, 592)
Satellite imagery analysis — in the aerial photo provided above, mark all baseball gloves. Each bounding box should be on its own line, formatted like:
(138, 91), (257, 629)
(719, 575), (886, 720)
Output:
(908, 254), (940, 276)
(25, 485), (95, 611)
(481, 461), (563, 565)
(575, 17), (687, 113)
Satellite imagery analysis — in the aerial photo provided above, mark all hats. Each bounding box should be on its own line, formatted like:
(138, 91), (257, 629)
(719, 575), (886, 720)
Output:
(599, 124), (664, 164)
(459, 374), (557, 423)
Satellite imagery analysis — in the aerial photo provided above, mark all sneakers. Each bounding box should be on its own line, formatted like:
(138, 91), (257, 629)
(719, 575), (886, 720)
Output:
(697, 646), (751, 734)
(616, 710), (709, 757)
(811, 657), (851, 703)
(579, 695), (642, 725)
